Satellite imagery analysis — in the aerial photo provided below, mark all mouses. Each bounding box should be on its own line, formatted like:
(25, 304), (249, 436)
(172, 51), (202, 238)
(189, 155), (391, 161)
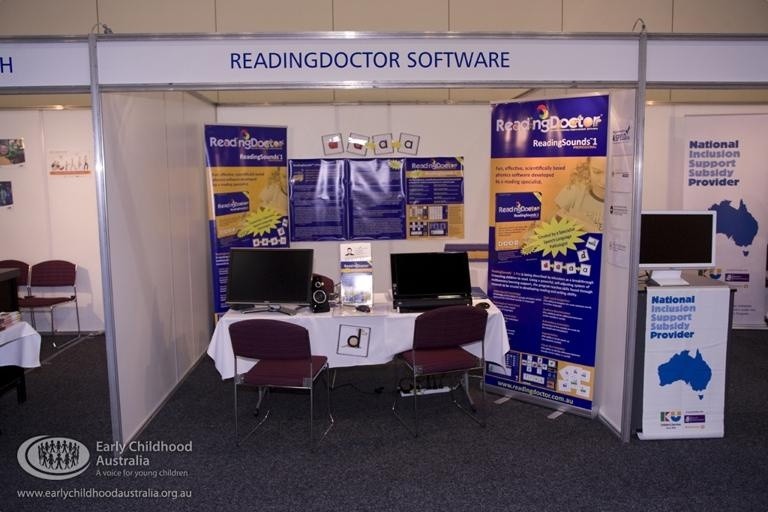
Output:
(476, 302), (491, 311)
(356, 305), (371, 313)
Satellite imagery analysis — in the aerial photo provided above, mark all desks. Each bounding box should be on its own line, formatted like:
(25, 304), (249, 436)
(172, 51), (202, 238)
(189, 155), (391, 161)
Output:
(631, 270), (737, 440)
(1, 320), (29, 406)
(215, 293), (503, 416)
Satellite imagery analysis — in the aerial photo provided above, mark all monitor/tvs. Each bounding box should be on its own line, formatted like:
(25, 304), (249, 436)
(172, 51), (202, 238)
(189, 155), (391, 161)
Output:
(224, 246), (314, 316)
(388, 252), (471, 312)
(639, 209), (717, 286)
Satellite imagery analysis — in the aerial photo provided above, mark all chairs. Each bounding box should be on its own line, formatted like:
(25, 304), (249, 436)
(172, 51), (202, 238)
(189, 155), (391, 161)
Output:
(392, 306), (489, 438)
(228, 319), (335, 453)
(0, 260), (82, 350)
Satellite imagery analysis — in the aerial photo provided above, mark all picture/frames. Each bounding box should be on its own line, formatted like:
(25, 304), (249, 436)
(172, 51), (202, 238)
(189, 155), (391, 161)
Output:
(321, 132), (420, 157)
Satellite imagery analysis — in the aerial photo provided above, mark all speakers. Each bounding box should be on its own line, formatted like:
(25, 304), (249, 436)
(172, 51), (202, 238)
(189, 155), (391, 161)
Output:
(310, 274), (331, 313)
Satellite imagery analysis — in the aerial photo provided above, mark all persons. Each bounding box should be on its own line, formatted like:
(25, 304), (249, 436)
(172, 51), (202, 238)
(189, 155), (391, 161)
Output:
(346, 248), (354, 256)
(0, 144), (12, 166)
